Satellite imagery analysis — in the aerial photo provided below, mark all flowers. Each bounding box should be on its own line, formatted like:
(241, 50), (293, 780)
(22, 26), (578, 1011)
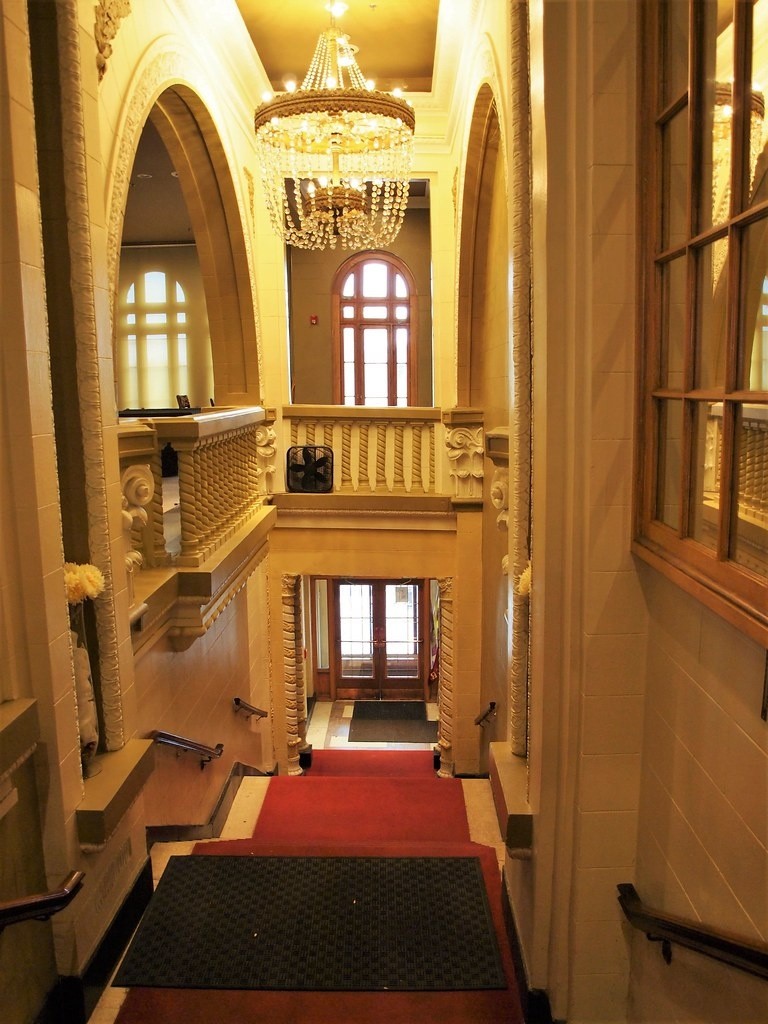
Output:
(516, 566), (533, 597)
(62, 560), (106, 606)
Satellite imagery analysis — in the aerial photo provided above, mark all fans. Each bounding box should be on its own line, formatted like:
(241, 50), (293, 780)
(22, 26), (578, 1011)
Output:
(287, 445), (333, 493)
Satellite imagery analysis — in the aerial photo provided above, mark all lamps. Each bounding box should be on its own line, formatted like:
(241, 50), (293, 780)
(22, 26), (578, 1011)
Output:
(710, 79), (766, 227)
(253, 1), (418, 251)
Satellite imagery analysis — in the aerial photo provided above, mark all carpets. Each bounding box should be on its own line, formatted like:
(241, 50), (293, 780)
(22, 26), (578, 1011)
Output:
(109, 855), (512, 992)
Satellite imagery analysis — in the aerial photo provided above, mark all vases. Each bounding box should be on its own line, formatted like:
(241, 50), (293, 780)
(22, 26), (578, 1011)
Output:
(67, 601), (104, 779)
